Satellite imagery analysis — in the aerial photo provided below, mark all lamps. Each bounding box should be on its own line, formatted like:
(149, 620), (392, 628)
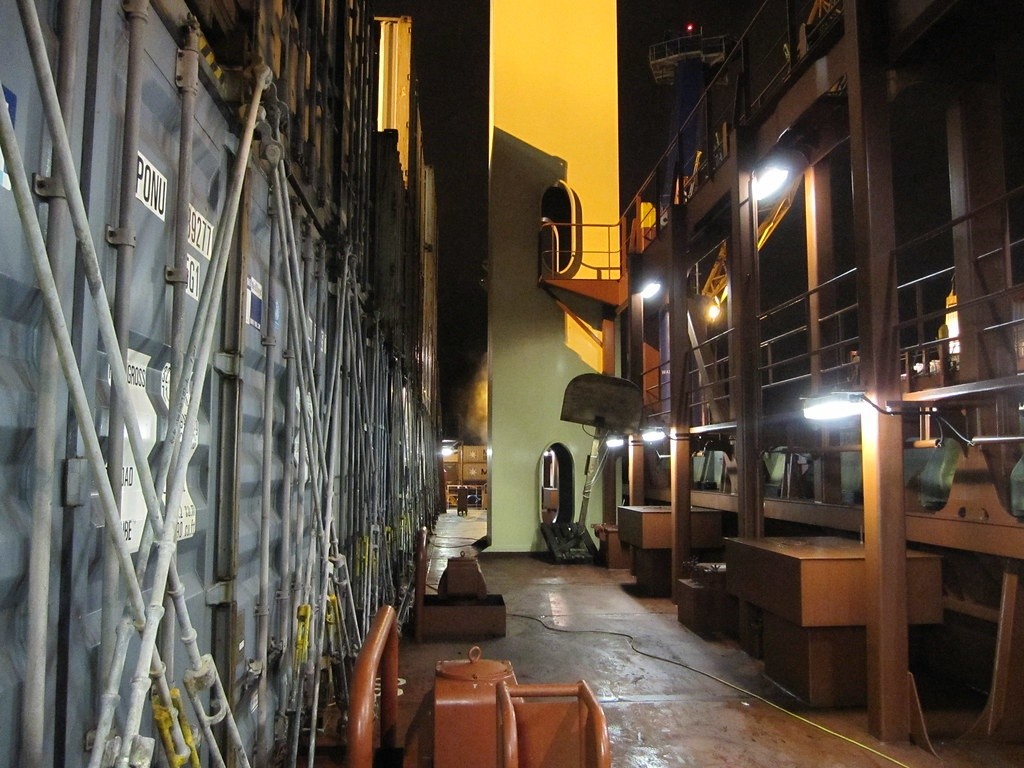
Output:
(799, 385), (976, 448)
